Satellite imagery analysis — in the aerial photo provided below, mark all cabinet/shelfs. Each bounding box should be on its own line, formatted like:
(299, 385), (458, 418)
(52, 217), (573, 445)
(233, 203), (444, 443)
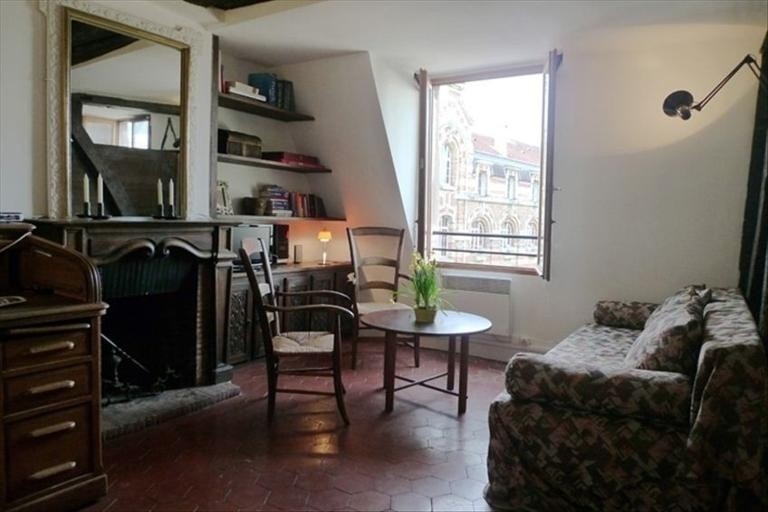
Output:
(209, 35), (354, 222)
(230, 263), (354, 367)
(0, 220), (111, 512)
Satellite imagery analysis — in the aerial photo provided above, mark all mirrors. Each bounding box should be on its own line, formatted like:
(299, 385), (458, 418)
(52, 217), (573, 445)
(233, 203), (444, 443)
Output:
(62, 5), (191, 219)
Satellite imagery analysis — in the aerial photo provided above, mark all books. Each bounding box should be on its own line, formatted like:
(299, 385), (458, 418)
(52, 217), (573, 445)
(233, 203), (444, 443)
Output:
(217, 63), (327, 218)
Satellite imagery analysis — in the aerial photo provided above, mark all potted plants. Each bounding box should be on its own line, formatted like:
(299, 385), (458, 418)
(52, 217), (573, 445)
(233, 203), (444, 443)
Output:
(389, 248), (459, 323)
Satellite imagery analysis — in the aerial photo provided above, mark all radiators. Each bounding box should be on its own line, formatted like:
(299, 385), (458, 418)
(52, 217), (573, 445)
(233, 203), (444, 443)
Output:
(443, 274), (511, 339)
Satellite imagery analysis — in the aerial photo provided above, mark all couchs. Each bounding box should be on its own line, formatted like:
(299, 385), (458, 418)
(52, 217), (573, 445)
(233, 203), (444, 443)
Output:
(483, 287), (768, 512)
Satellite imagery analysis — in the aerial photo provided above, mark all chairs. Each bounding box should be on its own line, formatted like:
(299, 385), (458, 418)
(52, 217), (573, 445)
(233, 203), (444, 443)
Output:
(346, 227), (420, 368)
(238, 238), (354, 426)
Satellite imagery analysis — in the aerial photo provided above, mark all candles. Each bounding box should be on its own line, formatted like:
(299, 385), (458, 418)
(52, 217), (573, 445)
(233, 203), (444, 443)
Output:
(83, 173), (91, 202)
(157, 178), (164, 205)
(169, 177), (175, 205)
(97, 173), (105, 203)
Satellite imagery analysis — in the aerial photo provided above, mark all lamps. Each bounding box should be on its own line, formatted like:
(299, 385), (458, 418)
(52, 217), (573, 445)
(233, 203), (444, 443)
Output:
(663, 54), (762, 120)
(318, 227), (332, 265)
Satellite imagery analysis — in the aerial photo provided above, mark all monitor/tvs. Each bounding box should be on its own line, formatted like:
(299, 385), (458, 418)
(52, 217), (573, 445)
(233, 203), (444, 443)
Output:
(227, 224), (273, 268)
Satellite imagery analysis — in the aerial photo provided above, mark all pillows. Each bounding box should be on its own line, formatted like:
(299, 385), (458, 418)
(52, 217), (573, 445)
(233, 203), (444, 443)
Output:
(623, 284), (711, 372)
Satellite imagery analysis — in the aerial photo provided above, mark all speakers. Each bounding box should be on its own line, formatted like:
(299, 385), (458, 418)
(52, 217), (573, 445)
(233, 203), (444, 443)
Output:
(294, 244), (303, 263)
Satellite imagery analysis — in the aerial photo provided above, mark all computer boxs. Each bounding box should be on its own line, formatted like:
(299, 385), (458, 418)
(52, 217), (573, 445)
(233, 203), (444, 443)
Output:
(273, 224), (289, 265)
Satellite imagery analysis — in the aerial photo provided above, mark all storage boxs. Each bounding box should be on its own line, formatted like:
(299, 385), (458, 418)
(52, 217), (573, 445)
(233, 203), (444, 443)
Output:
(218, 128), (262, 160)
